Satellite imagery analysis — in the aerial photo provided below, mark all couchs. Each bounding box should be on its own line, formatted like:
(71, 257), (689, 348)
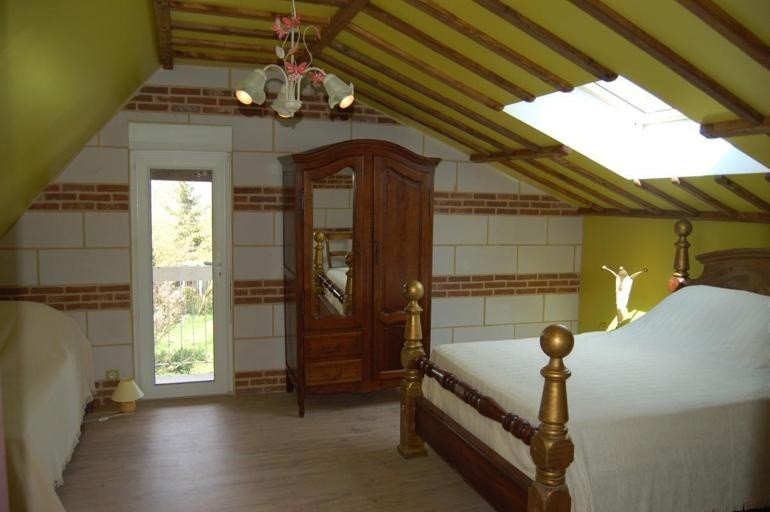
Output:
(0, 300), (95, 512)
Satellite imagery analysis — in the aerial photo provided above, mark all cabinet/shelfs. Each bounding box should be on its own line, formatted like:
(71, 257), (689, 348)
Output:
(277, 139), (442, 418)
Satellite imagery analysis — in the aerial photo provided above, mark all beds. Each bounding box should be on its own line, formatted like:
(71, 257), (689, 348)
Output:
(314, 231), (353, 317)
(398, 218), (770, 512)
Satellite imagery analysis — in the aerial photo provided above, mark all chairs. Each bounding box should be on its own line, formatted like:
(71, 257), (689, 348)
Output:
(324, 232), (354, 268)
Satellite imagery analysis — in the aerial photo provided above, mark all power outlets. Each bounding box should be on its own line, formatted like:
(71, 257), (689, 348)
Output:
(106, 370), (120, 382)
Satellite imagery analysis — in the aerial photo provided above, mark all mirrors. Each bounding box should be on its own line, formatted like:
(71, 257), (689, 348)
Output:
(314, 167), (354, 317)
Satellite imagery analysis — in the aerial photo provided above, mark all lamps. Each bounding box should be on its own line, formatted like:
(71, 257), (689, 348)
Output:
(111, 379), (144, 412)
(236, 0), (354, 118)
